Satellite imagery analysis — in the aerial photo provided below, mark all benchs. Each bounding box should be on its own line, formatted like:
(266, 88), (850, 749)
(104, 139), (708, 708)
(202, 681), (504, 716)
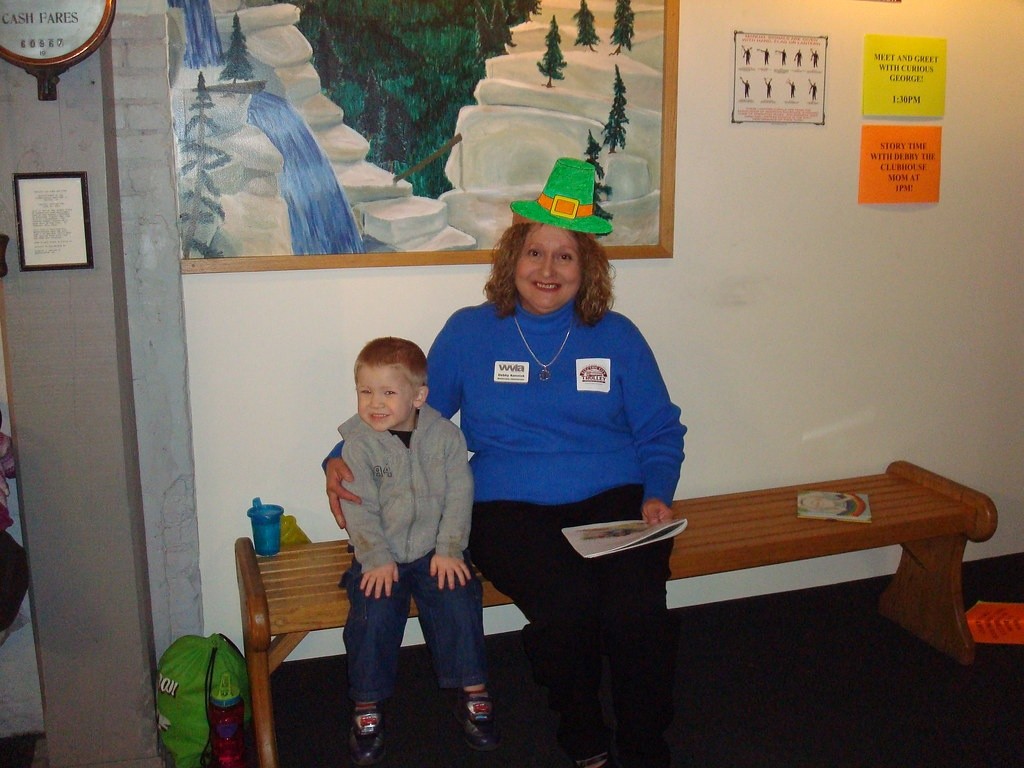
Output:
(234, 459), (998, 768)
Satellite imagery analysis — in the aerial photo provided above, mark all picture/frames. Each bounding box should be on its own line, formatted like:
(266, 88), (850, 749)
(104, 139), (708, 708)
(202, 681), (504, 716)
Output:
(166, 0), (680, 277)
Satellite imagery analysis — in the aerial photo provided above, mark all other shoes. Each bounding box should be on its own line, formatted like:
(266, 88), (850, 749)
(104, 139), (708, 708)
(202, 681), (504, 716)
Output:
(574, 751), (614, 768)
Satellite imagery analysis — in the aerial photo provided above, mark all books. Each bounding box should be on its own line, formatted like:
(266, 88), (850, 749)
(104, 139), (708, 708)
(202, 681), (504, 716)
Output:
(560, 518), (686, 559)
(794, 489), (872, 524)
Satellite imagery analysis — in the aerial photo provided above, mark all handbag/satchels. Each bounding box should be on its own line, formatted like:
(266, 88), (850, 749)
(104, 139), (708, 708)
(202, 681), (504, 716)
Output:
(156, 633), (251, 768)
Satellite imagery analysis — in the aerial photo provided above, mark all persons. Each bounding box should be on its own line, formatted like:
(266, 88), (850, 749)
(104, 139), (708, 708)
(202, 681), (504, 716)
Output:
(338, 336), (500, 768)
(322, 217), (686, 768)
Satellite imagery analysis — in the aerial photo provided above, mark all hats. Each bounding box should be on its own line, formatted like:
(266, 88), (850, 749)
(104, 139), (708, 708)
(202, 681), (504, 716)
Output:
(510, 157), (613, 234)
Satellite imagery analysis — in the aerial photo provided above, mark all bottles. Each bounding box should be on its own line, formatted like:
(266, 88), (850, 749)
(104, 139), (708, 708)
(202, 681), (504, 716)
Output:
(208, 687), (245, 768)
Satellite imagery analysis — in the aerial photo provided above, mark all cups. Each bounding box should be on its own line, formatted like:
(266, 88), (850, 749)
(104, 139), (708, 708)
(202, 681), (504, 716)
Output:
(246, 497), (284, 556)
(280, 515), (312, 546)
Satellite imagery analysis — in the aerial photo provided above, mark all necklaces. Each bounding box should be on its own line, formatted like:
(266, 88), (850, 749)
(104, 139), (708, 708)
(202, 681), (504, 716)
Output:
(514, 314), (573, 380)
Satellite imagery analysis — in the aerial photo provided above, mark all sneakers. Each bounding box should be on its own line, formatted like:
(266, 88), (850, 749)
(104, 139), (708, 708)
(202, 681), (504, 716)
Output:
(462, 689), (504, 751)
(346, 700), (387, 766)
